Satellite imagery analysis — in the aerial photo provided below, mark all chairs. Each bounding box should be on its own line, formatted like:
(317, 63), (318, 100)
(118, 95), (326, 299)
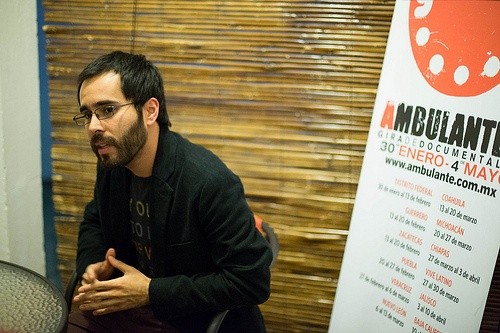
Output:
(65, 216), (280, 333)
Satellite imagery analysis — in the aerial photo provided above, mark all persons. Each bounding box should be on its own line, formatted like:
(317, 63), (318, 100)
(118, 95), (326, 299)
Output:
(72, 50), (273, 333)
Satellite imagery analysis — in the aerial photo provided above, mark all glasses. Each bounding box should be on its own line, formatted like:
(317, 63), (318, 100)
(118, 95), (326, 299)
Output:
(73, 102), (134, 125)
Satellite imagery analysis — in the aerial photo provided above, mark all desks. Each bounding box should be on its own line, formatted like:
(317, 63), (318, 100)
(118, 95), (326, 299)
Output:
(0, 260), (68, 333)
